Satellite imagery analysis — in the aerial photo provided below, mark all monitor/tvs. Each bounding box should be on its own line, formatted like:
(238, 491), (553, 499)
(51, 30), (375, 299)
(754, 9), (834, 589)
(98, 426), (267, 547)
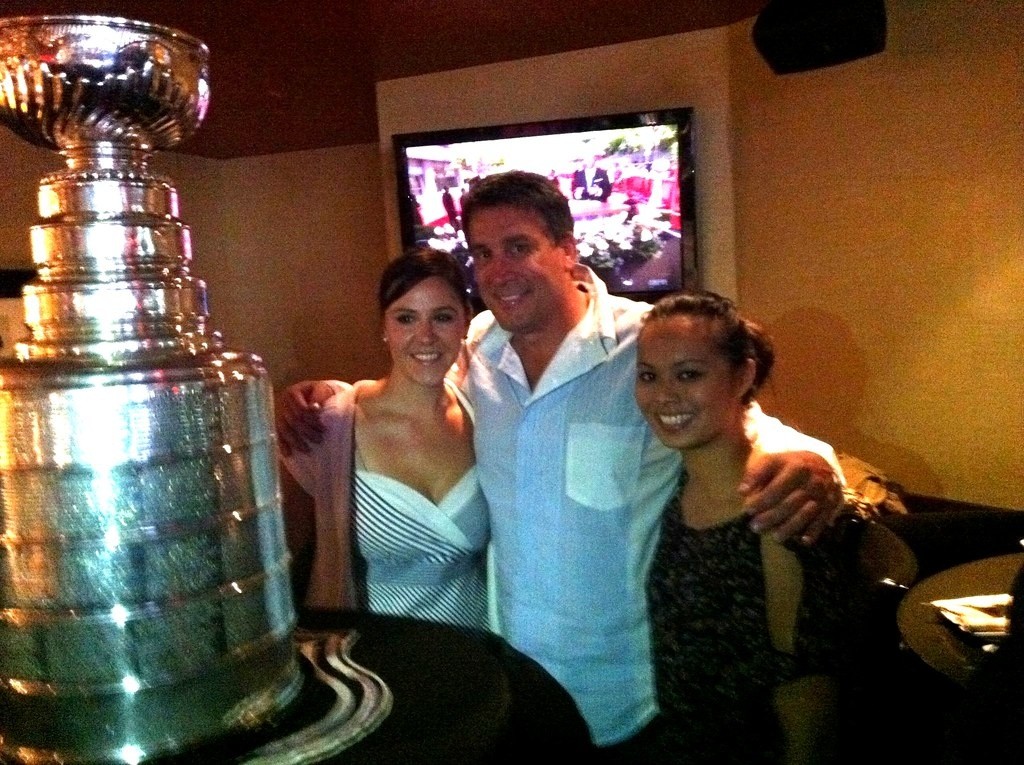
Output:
(393, 106), (702, 306)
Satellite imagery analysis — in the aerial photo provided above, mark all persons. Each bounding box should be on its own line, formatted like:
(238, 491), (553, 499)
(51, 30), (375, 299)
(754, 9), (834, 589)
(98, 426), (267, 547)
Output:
(442, 186), (460, 232)
(572, 151), (611, 201)
(276, 172), (844, 765)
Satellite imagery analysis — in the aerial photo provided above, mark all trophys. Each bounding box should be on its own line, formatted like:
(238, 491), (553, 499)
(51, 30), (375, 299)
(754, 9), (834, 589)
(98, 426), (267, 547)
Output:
(0, 12), (338, 764)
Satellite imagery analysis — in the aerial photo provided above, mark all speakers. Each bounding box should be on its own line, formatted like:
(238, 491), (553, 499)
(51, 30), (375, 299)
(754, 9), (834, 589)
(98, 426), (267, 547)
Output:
(752, 0), (887, 76)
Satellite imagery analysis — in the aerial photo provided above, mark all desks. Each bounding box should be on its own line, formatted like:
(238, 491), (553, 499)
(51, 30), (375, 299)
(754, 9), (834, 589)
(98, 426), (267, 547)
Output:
(899, 553), (1024, 680)
(0, 606), (599, 765)
